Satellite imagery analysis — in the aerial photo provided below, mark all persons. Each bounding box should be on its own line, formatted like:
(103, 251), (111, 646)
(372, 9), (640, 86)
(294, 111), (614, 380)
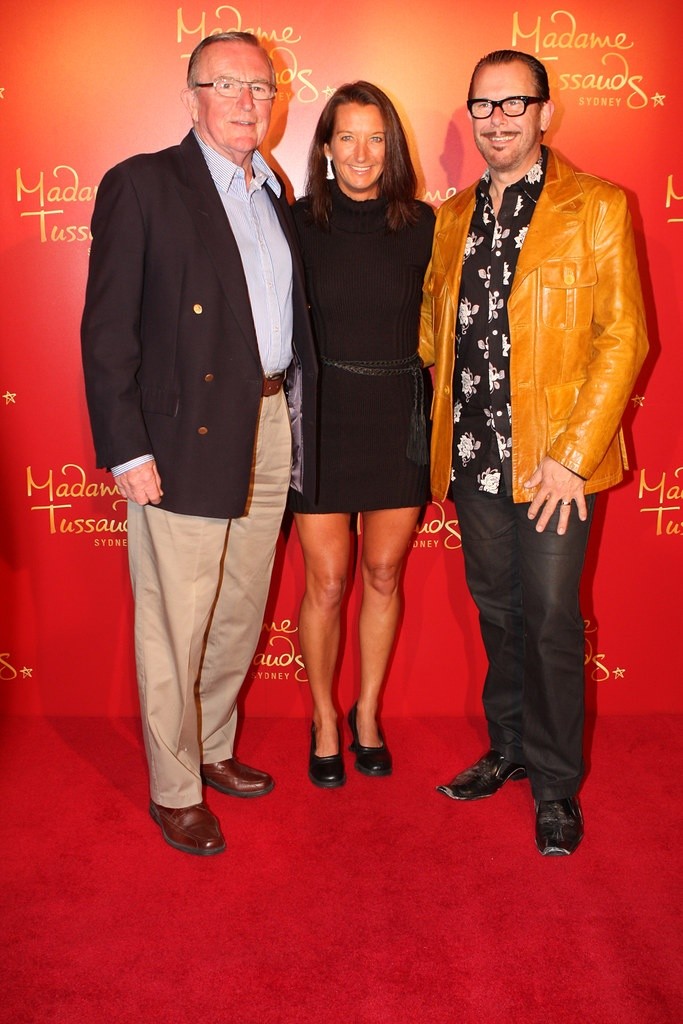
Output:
(416, 47), (649, 854)
(288, 80), (438, 788)
(78, 32), (321, 858)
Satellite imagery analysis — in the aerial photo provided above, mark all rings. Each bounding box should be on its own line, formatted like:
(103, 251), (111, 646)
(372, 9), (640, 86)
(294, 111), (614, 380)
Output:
(561, 501), (571, 504)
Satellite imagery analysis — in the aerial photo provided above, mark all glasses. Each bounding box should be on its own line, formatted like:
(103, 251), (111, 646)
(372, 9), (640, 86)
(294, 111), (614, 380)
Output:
(467, 95), (543, 119)
(195, 76), (277, 100)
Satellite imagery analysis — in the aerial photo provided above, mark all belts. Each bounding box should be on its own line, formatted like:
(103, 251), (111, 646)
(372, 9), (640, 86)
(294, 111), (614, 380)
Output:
(262, 370), (287, 396)
(317, 350), (432, 468)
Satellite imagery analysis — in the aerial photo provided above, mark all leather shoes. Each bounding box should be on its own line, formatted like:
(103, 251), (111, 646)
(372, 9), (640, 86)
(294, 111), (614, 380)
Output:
(200, 757), (274, 797)
(435, 749), (528, 800)
(532, 797), (584, 856)
(148, 798), (225, 855)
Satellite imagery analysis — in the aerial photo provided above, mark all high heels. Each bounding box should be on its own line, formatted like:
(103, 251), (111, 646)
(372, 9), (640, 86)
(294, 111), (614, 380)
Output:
(308, 719), (346, 788)
(348, 701), (393, 776)
